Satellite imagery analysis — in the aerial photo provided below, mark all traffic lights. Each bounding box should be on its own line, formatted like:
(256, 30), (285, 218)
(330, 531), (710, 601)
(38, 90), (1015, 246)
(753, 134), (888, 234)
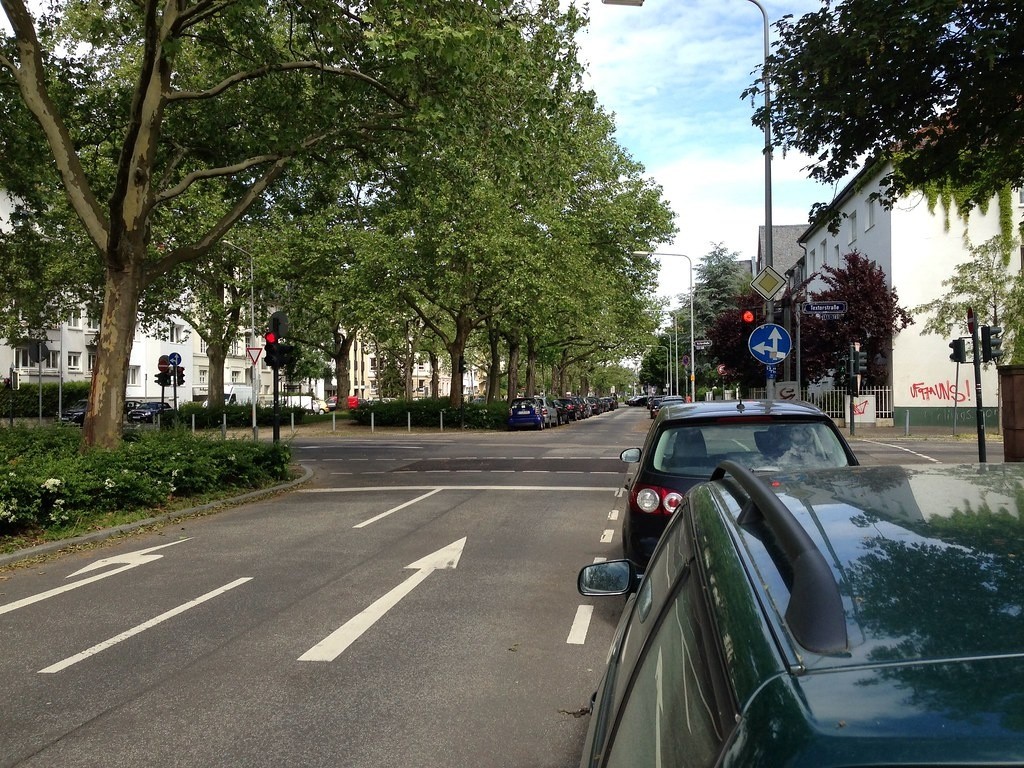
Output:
(853, 350), (868, 375)
(154, 371), (171, 389)
(263, 333), (279, 368)
(742, 309), (758, 323)
(278, 344), (296, 368)
(949, 339), (966, 364)
(173, 366), (185, 386)
(982, 326), (1004, 364)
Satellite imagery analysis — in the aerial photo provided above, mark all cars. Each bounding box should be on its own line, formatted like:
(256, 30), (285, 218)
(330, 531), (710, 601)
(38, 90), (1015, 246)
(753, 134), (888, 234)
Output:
(124, 400), (143, 414)
(312, 394), (420, 417)
(54, 399), (88, 427)
(620, 399), (867, 610)
(127, 402), (174, 424)
(624, 393), (688, 420)
(506, 394), (618, 432)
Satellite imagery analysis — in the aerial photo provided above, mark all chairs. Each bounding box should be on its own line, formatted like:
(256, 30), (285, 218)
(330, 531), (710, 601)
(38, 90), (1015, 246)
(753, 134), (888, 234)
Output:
(752, 426), (807, 466)
(666, 429), (710, 474)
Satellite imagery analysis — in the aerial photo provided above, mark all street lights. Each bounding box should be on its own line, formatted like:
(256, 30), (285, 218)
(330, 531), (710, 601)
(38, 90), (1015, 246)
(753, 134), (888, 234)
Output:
(222, 239), (257, 433)
(649, 330), (673, 398)
(632, 249), (699, 405)
(645, 345), (670, 396)
(594, 0), (778, 399)
(642, 309), (679, 397)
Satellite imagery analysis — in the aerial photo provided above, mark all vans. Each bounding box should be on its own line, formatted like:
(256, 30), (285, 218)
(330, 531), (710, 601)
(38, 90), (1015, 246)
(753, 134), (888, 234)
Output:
(269, 394), (315, 415)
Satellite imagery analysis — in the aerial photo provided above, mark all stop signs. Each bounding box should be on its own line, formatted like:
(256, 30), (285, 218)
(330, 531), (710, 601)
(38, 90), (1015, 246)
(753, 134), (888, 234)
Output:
(966, 308), (974, 333)
(157, 359), (168, 372)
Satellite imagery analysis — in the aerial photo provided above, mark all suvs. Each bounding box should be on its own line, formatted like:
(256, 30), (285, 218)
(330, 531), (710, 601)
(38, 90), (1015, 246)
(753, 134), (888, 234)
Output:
(576, 459), (1024, 768)
